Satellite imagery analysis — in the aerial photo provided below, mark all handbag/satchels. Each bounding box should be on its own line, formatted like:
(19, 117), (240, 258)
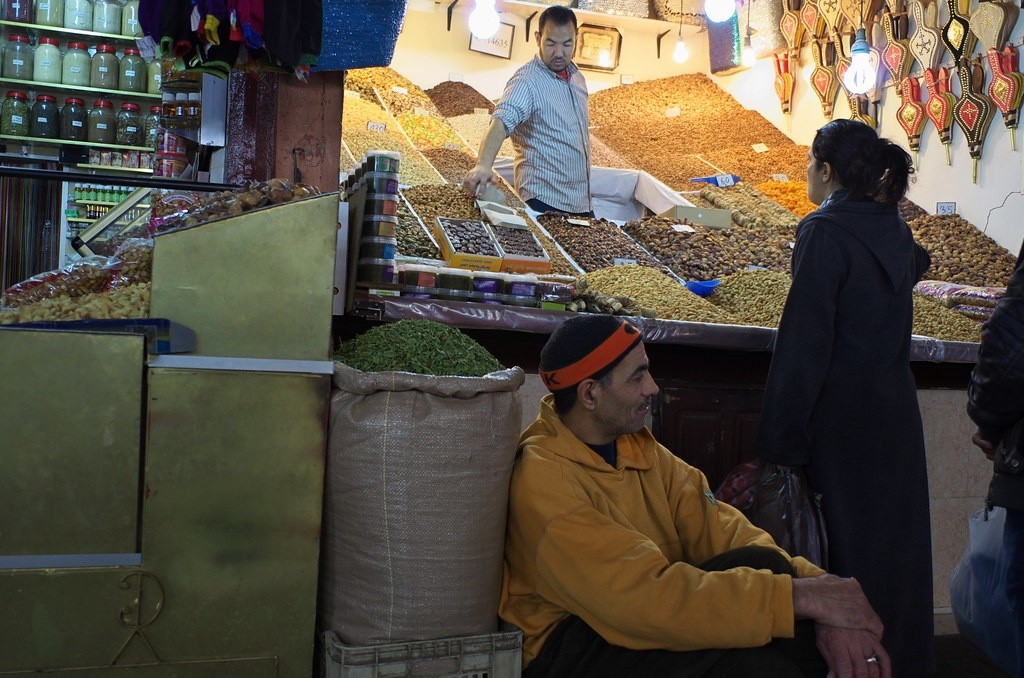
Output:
(716, 461), (832, 574)
(950, 502), (1023, 673)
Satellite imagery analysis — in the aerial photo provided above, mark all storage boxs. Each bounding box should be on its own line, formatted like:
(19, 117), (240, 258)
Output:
(318, 629), (523, 678)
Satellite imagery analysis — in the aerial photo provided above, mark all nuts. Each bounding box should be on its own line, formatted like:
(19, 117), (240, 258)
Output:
(0, 245), (152, 324)
(536, 211), (797, 281)
(898, 197), (1017, 287)
(151, 178), (321, 227)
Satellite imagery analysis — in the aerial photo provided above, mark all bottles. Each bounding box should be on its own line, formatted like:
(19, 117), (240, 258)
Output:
(0, 0), (201, 149)
(74, 184), (150, 205)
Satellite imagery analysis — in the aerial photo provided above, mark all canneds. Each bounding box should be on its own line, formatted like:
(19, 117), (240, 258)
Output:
(0, 0), (164, 147)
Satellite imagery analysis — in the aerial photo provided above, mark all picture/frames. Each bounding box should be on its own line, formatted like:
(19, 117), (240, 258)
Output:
(572, 23), (623, 73)
(469, 21), (515, 59)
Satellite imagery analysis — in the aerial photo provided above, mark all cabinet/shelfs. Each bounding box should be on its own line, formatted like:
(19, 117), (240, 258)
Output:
(0, 0), (233, 268)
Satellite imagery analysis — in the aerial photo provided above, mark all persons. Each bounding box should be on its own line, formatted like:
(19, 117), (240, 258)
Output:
(498, 315), (891, 678)
(967, 242), (1024, 678)
(756, 120), (933, 678)
(462, 6), (595, 218)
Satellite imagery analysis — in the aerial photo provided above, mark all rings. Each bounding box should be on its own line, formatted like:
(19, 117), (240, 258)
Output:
(866, 655), (880, 664)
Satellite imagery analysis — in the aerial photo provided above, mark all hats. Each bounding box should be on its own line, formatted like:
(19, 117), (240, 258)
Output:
(539, 311), (642, 396)
